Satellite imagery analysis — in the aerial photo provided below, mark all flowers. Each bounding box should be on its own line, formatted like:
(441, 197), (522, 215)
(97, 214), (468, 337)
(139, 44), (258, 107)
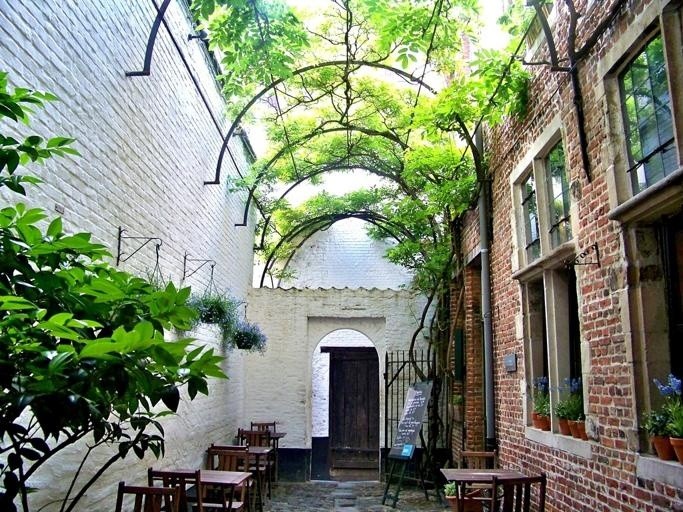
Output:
(639, 373), (683, 438)
(527, 376), (585, 421)
(186, 286), (267, 357)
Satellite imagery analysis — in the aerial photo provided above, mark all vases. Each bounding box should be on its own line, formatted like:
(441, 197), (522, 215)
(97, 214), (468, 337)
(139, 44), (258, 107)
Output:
(653, 436), (683, 466)
(531, 411), (587, 441)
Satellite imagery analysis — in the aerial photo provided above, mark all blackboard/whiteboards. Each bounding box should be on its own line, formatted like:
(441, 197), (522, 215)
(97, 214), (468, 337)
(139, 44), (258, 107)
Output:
(388, 381), (434, 460)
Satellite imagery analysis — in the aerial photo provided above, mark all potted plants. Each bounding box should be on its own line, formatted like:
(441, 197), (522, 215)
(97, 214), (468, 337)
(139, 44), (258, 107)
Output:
(443, 481), (483, 512)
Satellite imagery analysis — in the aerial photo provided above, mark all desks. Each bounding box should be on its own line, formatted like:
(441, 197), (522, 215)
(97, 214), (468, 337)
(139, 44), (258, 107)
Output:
(440, 467), (528, 512)
(236, 430), (287, 480)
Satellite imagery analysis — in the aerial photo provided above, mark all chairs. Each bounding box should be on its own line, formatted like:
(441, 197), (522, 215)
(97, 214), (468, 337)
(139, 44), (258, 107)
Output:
(117, 420), (276, 511)
(458, 447), (547, 512)
